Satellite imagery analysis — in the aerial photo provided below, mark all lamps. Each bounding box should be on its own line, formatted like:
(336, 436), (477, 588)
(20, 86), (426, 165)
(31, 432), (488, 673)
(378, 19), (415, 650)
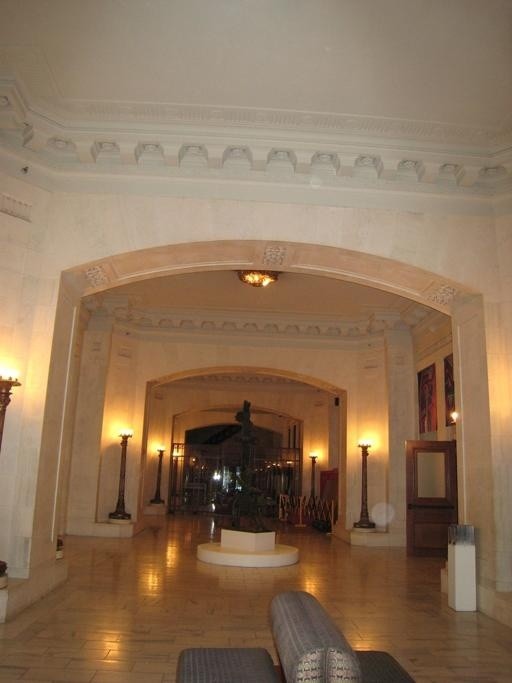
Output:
(237, 269), (278, 287)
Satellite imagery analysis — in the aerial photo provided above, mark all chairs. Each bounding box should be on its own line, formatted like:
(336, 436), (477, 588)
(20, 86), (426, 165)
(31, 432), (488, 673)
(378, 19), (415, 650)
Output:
(177, 591), (326, 683)
(298, 577), (415, 683)
